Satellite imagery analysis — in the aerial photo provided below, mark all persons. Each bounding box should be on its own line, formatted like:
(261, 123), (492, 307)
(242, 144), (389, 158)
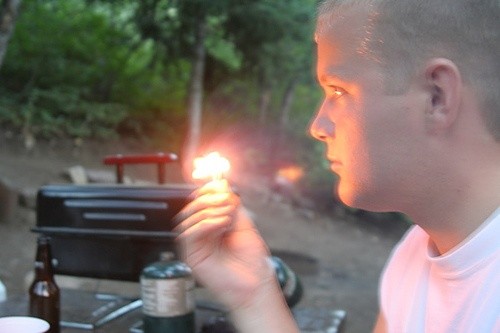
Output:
(168, 0), (500, 333)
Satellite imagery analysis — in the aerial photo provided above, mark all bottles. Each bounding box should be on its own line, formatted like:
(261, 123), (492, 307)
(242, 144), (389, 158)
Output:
(29, 236), (60, 333)
(139, 251), (195, 333)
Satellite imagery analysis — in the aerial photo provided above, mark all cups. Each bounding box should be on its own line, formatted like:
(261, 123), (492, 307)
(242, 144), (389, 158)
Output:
(0, 316), (51, 333)
